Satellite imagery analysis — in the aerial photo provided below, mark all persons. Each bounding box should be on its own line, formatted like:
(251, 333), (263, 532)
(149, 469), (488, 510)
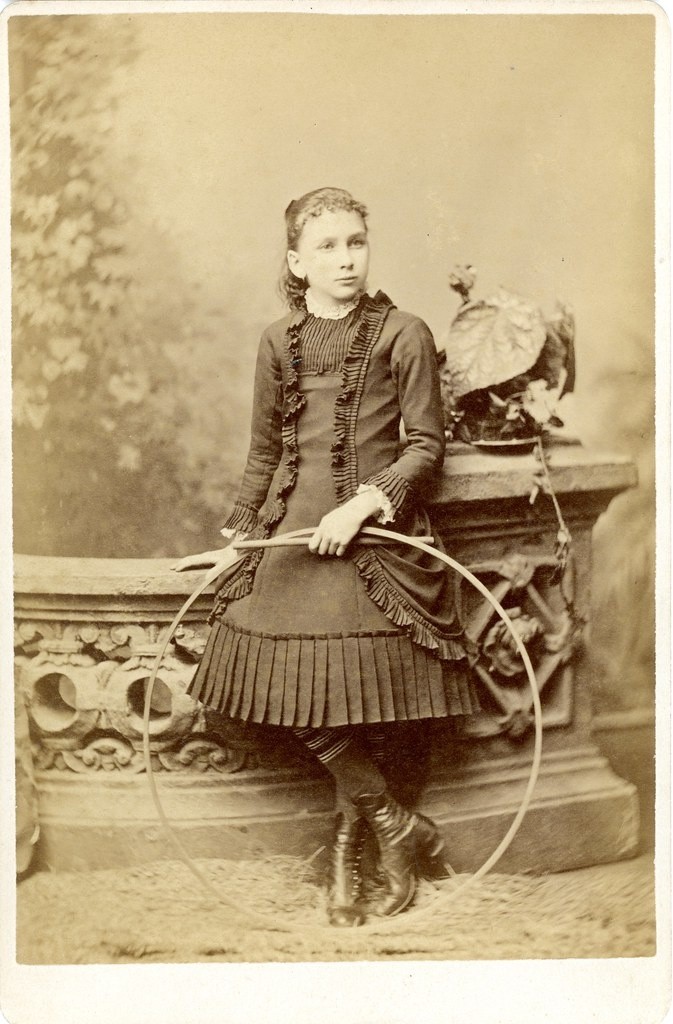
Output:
(169, 185), (481, 924)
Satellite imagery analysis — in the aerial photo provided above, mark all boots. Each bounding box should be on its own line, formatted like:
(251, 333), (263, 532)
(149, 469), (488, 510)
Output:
(328, 780), (446, 930)
